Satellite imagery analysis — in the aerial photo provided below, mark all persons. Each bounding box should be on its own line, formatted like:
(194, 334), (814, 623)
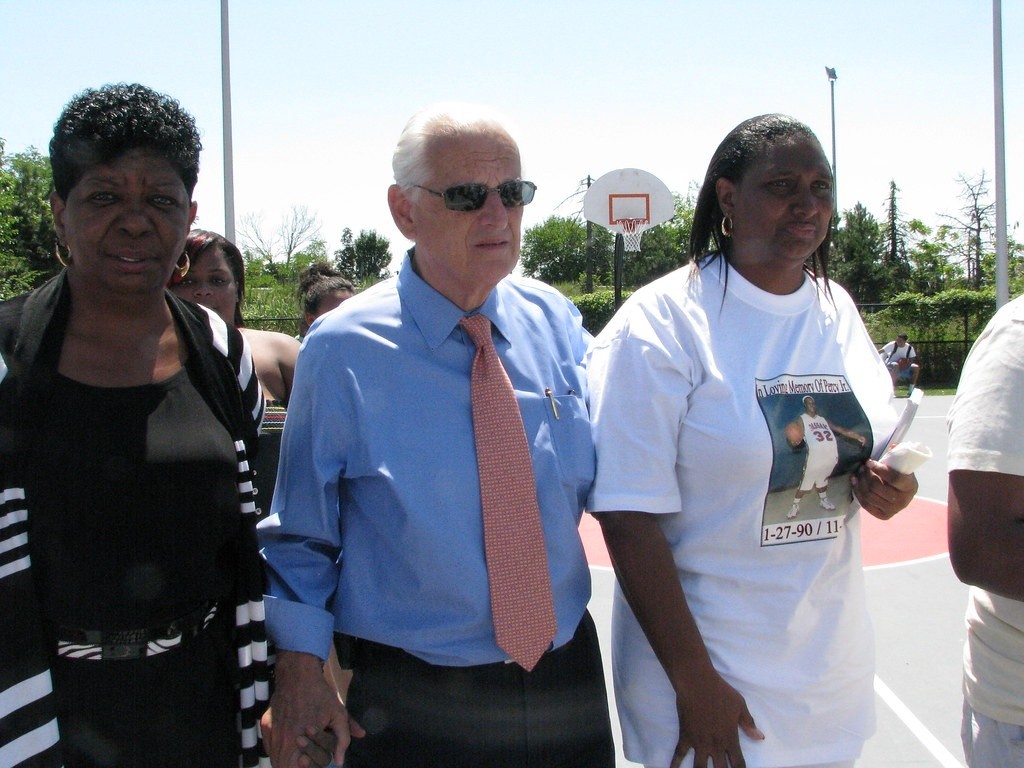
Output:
(878, 332), (919, 394)
(0, 81), (365, 767)
(589, 113), (914, 768)
(298, 260), (355, 325)
(785, 394), (867, 518)
(171, 229), (303, 520)
(949, 296), (1024, 768)
(256, 105), (616, 767)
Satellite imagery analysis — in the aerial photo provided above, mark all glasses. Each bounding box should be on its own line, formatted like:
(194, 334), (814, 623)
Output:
(411, 182), (538, 212)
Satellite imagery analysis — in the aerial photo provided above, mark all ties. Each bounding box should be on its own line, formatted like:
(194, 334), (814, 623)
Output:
(458, 312), (556, 672)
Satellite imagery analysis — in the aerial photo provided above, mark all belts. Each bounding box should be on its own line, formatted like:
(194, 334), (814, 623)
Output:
(54, 598), (227, 660)
